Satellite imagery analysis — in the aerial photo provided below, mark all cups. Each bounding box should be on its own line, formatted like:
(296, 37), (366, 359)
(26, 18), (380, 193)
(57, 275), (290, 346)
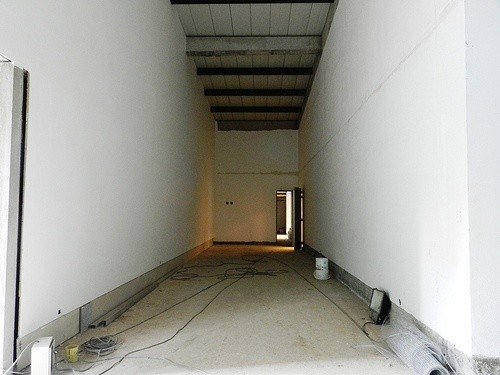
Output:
(64, 344), (78, 363)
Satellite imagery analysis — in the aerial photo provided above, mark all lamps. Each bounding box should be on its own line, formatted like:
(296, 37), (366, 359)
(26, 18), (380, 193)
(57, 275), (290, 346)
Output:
(368, 287), (392, 324)
